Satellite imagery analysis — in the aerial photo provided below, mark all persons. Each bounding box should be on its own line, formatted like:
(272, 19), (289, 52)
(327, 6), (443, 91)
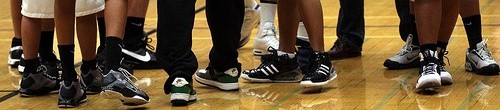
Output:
(9, 0), (500, 107)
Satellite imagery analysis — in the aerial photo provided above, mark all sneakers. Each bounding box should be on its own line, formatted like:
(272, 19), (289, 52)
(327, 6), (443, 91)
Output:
(57, 78), (87, 107)
(437, 47), (453, 85)
(101, 68), (150, 106)
(241, 47), (302, 82)
(416, 49), (441, 92)
(300, 47), (338, 87)
(254, 22), (280, 55)
(8, 45), (23, 66)
(323, 39), (362, 59)
(39, 53), (63, 75)
(169, 77), (197, 103)
(97, 46), (135, 73)
(79, 64), (104, 94)
(17, 53), (26, 73)
(194, 68), (240, 91)
(122, 32), (159, 68)
(239, 0), (261, 47)
(465, 39), (500, 74)
(17, 65), (60, 97)
(382, 34), (421, 68)
